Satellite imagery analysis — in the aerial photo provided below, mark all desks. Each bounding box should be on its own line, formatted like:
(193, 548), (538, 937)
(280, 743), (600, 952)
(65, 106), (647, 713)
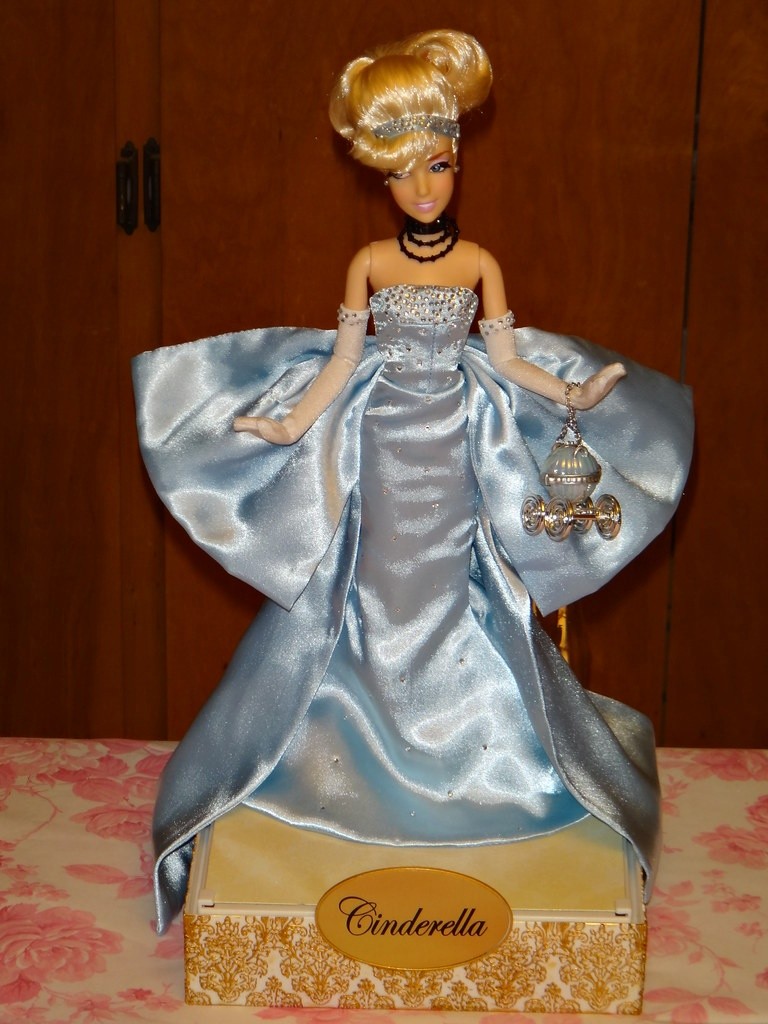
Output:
(0, 740), (767, 1024)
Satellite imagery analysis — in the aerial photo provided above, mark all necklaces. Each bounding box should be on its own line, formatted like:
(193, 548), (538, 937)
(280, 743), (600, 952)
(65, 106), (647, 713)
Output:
(396, 212), (461, 264)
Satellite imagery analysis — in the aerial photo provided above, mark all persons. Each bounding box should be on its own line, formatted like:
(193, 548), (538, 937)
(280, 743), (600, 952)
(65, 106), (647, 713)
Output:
(130, 28), (695, 937)
